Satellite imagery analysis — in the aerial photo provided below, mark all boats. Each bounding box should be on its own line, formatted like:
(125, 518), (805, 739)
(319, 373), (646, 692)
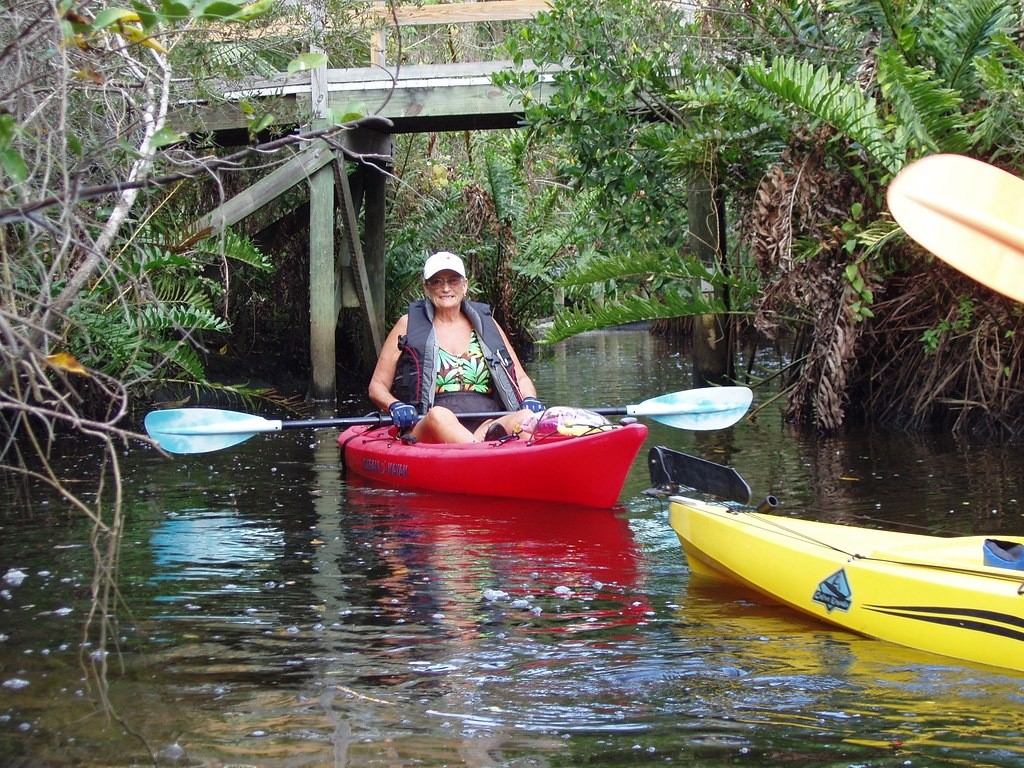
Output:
(665, 493), (1024, 674)
(359, 414), (648, 511)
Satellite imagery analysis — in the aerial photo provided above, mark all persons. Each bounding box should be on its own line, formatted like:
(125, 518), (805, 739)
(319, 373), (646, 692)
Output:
(369, 251), (547, 444)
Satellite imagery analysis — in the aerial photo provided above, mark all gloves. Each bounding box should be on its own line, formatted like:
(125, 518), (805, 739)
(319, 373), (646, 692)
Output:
(520, 397), (547, 413)
(388, 401), (419, 432)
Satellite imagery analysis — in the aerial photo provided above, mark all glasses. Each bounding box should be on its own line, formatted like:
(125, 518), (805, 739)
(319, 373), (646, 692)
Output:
(424, 277), (464, 290)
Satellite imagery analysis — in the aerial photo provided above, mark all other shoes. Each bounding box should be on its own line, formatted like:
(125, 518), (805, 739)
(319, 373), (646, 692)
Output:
(483, 422), (508, 442)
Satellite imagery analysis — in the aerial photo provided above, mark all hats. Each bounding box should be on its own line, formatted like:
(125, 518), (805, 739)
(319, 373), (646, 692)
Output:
(423, 251), (466, 279)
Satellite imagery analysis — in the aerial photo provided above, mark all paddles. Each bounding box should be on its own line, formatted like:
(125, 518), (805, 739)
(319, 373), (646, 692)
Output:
(142, 386), (756, 457)
(883, 154), (1023, 306)
